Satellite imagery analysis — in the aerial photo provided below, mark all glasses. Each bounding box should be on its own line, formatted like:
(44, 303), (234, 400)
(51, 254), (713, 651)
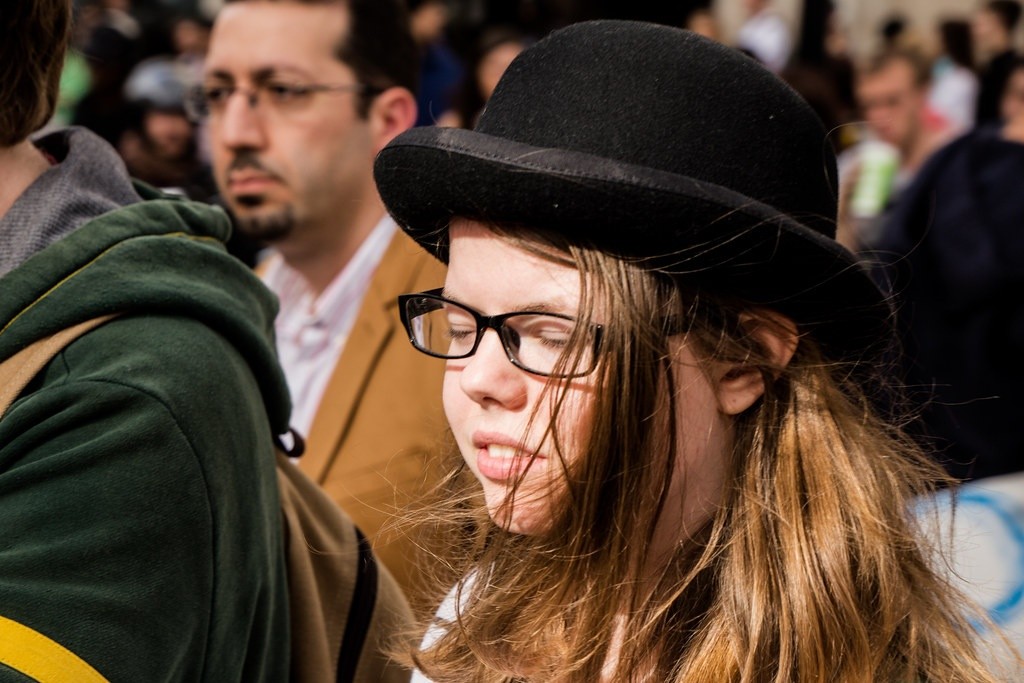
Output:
(396, 285), (705, 380)
(184, 78), (367, 124)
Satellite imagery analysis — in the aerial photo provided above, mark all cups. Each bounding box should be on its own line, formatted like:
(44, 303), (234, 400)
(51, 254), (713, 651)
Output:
(849, 138), (900, 221)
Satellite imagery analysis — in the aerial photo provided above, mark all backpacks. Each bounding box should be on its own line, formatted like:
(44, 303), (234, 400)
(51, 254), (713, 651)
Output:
(0, 313), (418, 681)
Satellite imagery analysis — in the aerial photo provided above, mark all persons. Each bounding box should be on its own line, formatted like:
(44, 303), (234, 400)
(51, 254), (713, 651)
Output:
(371, 19), (1024, 682)
(399, 1), (536, 135)
(1, 1), (300, 683)
(682, 1), (1024, 683)
(200, 0), (506, 636)
(49, 0), (266, 273)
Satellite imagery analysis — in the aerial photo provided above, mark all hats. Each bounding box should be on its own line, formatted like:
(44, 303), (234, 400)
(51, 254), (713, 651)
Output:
(372, 18), (896, 365)
